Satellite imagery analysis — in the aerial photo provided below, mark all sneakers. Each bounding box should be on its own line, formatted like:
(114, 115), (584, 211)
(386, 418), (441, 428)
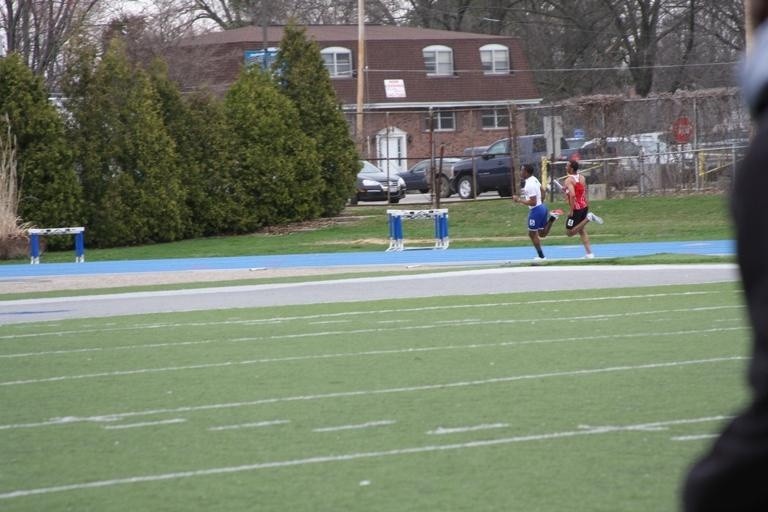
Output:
(579, 253), (595, 260)
(586, 211), (604, 226)
(533, 256), (547, 262)
(549, 209), (565, 220)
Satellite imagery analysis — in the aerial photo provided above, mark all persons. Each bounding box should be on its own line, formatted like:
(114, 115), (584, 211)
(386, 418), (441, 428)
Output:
(563, 160), (605, 258)
(675, 24), (768, 512)
(512, 163), (561, 260)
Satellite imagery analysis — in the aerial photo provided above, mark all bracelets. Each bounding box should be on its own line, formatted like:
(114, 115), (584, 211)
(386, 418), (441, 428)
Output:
(517, 198), (521, 202)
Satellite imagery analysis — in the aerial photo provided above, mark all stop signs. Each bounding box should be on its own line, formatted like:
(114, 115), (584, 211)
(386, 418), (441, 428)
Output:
(672, 116), (691, 143)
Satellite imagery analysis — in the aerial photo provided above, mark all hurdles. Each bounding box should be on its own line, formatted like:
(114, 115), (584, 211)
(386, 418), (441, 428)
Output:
(26, 226), (86, 265)
(386, 209), (448, 250)
